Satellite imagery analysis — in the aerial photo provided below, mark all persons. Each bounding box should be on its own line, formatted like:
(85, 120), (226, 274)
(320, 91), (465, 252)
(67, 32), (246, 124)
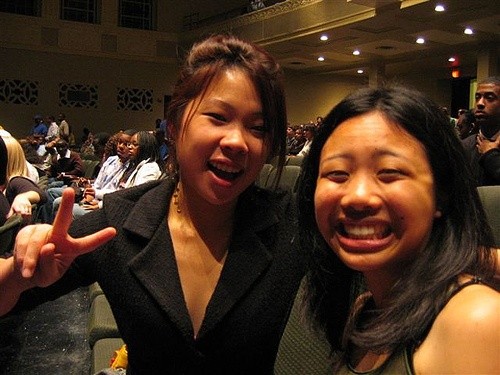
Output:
(0, 77), (500, 228)
(271, 84), (500, 375)
(0, 35), (500, 375)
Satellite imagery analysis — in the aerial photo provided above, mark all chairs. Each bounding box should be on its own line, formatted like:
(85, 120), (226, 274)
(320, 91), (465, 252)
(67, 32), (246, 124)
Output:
(0, 156), (500, 375)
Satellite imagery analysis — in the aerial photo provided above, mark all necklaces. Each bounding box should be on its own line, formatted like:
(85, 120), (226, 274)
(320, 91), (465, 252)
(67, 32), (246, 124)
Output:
(174, 182), (208, 240)
